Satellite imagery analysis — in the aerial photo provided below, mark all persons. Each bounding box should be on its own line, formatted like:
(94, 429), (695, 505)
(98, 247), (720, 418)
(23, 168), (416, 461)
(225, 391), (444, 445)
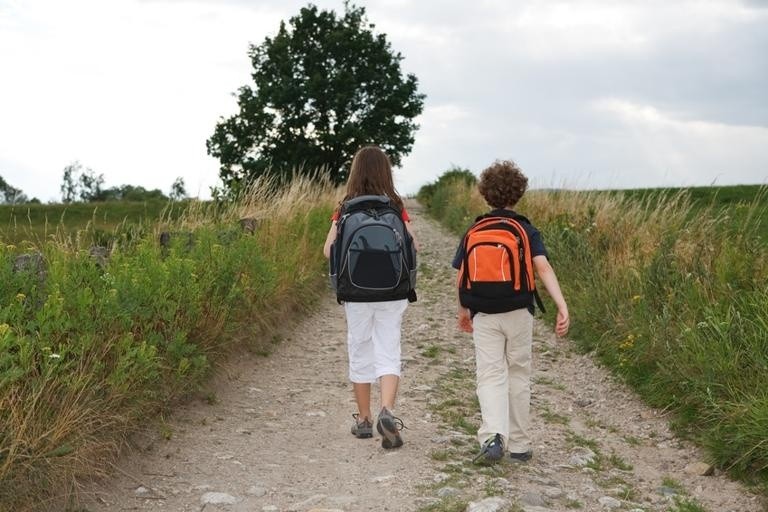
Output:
(322, 148), (420, 449)
(453, 153), (572, 466)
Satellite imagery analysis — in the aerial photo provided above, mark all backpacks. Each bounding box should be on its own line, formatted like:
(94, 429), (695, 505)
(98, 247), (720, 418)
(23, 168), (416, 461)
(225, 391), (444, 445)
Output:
(329, 193), (417, 305)
(459, 213), (546, 315)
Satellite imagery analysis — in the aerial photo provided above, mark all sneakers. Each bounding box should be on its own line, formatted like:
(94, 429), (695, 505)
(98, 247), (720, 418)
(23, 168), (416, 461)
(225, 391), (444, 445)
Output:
(377, 406), (402, 449)
(473, 433), (504, 465)
(351, 416), (372, 438)
